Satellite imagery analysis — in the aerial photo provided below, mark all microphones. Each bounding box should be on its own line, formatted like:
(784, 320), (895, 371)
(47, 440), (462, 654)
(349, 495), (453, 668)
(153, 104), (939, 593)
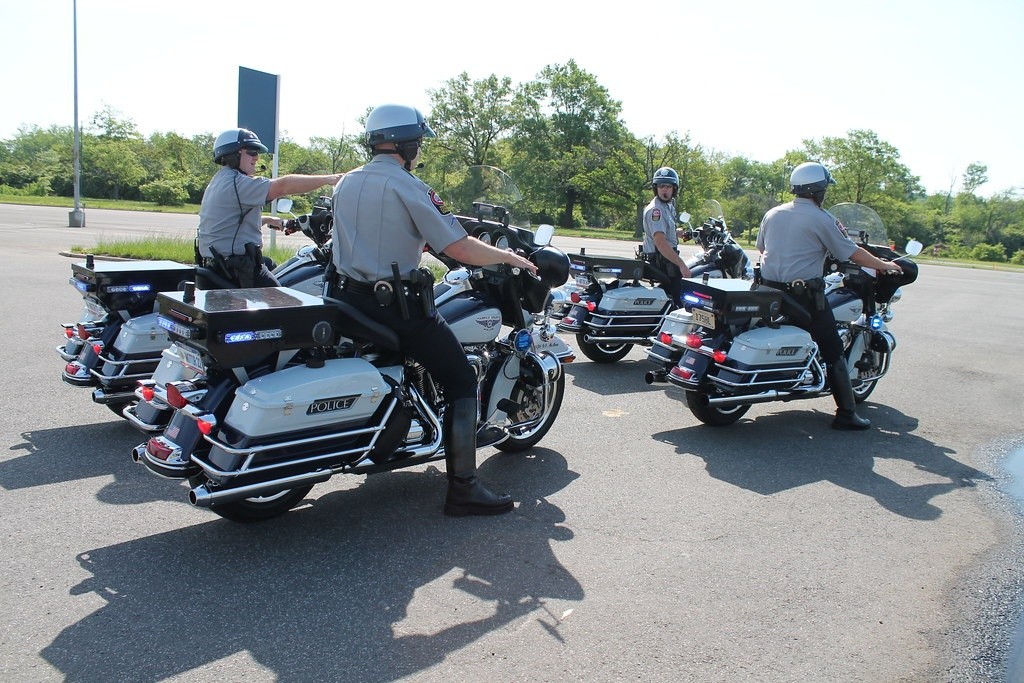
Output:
(663, 194), (672, 199)
(414, 163), (424, 169)
(256, 165), (267, 173)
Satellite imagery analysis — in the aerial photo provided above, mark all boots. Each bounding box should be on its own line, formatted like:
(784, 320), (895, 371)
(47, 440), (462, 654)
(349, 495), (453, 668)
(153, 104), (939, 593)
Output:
(826, 357), (870, 430)
(442, 397), (514, 516)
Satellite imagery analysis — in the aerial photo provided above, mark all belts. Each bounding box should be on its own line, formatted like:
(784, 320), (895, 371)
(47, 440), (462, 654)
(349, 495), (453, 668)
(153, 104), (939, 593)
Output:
(334, 273), (411, 298)
(762, 278), (792, 293)
(202, 257), (216, 267)
(642, 247), (677, 260)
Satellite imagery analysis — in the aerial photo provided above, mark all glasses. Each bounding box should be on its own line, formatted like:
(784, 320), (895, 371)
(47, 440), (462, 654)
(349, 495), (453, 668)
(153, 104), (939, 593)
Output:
(415, 137), (423, 147)
(656, 185), (670, 189)
(241, 147), (260, 156)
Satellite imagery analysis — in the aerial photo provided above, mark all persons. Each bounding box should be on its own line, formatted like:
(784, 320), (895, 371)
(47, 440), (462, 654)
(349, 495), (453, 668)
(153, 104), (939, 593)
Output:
(643, 167), (691, 309)
(196, 128), (345, 288)
(756, 162), (902, 430)
(324, 105), (539, 514)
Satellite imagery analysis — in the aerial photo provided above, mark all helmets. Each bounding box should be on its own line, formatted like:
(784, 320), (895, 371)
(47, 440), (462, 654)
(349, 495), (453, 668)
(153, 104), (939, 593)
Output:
(365, 104), (436, 146)
(212, 128), (268, 164)
(652, 167), (679, 198)
(789, 162), (838, 194)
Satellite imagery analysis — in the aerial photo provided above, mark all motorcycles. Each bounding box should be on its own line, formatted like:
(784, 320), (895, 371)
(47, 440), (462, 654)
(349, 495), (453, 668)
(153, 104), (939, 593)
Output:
(644, 201), (924, 427)
(122, 164), (576, 527)
(547, 198), (755, 366)
(56, 169), (341, 420)
